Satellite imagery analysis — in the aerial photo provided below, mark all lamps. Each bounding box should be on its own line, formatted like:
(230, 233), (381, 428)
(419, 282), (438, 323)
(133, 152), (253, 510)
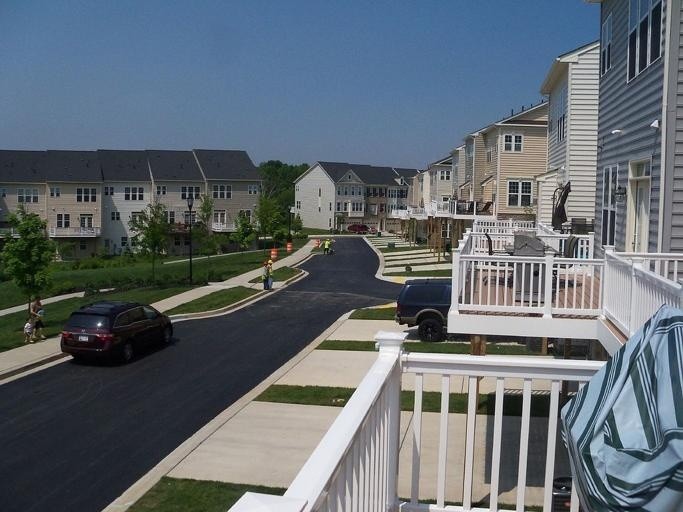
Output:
(486, 218), (595, 303)
(456, 200), (493, 215)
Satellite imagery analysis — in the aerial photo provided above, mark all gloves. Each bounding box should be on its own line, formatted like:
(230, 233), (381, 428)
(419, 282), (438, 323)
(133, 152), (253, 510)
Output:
(551, 477), (581, 512)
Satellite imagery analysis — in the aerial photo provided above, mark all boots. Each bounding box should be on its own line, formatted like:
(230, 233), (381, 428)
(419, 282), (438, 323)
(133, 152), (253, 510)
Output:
(395, 278), (452, 342)
(347, 224), (368, 235)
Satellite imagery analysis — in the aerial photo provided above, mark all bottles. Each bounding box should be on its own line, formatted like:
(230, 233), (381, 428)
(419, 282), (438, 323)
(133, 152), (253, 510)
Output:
(264, 262), (268, 265)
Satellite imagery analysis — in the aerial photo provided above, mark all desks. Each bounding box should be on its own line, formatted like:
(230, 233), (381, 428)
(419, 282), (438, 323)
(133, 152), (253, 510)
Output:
(287, 204), (292, 243)
(187, 193), (194, 285)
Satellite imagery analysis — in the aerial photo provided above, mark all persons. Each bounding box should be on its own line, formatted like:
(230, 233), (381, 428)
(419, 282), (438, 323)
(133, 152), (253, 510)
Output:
(267, 259), (274, 290)
(322, 238), (331, 254)
(28, 296), (47, 340)
(262, 261), (269, 290)
(22, 317), (37, 343)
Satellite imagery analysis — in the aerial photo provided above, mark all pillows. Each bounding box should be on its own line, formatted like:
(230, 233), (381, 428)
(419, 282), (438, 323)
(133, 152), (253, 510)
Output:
(268, 260), (273, 264)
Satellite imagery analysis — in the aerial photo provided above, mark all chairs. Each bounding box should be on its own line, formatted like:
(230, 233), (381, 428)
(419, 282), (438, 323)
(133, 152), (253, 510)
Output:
(30, 334), (46, 342)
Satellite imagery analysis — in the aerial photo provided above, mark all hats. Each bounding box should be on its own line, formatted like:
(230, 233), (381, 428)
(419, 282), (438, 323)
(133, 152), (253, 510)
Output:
(60, 300), (174, 362)
(368, 227), (376, 234)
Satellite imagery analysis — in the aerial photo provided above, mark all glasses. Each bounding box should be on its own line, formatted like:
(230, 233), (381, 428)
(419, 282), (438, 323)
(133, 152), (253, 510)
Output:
(614, 185), (626, 202)
